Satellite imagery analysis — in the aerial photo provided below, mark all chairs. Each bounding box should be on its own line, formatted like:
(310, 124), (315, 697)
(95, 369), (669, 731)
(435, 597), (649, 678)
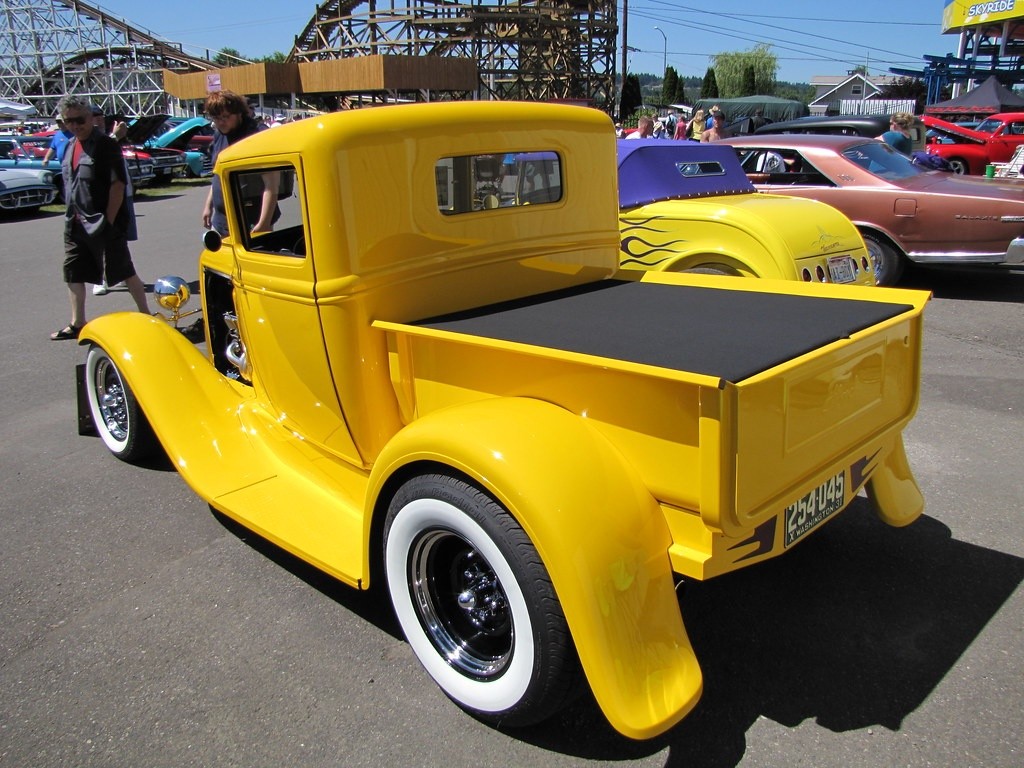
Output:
(983, 144), (1024, 177)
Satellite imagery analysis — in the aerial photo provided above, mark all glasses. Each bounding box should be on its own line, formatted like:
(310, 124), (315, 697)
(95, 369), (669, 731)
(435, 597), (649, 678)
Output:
(63, 112), (89, 129)
(889, 122), (894, 125)
(212, 113), (232, 123)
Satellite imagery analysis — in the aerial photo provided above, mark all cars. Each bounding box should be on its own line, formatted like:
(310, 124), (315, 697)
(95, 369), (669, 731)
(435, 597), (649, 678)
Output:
(0, 114), (215, 218)
(753, 113), (1024, 175)
(700, 134), (1024, 285)
(615, 139), (876, 288)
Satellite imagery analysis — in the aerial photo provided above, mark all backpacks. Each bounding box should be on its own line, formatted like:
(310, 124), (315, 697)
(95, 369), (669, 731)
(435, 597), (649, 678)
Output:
(245, 119), (293, 200)
(667, 115), (676, 135)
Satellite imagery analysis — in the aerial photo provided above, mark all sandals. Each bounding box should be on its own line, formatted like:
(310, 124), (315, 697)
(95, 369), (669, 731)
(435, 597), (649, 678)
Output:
(50, 324), (82, 340)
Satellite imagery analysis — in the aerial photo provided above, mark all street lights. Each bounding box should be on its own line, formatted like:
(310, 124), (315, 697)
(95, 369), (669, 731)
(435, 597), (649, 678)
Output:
(654, 26), (667, 79)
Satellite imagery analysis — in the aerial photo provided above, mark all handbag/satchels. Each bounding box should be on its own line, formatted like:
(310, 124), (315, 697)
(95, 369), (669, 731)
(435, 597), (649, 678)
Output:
(685, 122), (693, 137)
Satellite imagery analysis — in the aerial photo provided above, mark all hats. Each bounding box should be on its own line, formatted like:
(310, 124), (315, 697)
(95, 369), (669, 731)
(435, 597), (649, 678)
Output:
(615, 123), (621, 127)
(708, 105), (720, 115)
(713, 112), (725, 121)
(55, 114), (63, 121)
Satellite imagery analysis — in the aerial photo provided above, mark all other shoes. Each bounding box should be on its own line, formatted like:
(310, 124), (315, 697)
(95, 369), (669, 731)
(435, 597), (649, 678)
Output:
(93, 280), (107, 294)
(115, 281), (127, 287)
(181, 318), (205, 341)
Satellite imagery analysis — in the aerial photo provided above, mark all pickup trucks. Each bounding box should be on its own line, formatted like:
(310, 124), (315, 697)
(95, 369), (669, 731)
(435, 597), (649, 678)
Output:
(76, 101), (932, 742)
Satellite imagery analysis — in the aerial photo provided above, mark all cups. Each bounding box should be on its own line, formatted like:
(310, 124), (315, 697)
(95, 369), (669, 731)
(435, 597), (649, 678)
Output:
(986, 165), (996, 178)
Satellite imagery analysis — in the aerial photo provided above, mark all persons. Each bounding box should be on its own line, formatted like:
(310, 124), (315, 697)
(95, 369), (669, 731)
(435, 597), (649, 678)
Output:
(674, 115), (687, 140)
(42, 95), (151, 340)
(753, 108), (766, 129)
(665, 110), (678, 138)
(699, 111), (729, 143)
(248, 104), (286, 131)
(687, 110), (706, 142)
(527, 160), (554, 190)
(615, 122), (625, 139)
(41, 122), (51, 132)
(203, 88), (282, 239)
(625, 116), (654, 139)
(652, 113), (665, 139)
(756, 151), (786, 173)
(29, 123), (33, 133)
(18, 121), (25, 133)
(875, 113), (914, 154)
(707, 105), (726, 129)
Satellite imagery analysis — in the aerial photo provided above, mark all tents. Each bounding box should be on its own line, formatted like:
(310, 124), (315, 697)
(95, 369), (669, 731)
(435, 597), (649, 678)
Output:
(926, 75), (1024, 122)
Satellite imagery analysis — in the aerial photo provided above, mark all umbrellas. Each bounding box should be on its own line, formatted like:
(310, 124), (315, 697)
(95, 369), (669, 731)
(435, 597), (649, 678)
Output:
(0, 99), (36, 116)
